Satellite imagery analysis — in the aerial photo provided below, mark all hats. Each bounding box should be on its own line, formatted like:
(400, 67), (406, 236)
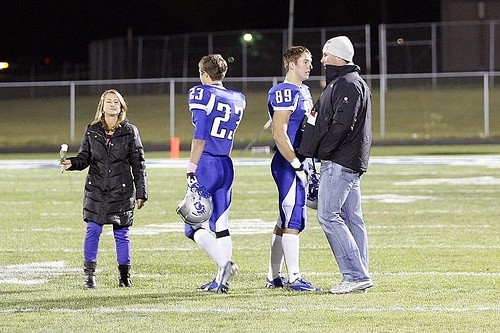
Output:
(323, 36), (354, 62)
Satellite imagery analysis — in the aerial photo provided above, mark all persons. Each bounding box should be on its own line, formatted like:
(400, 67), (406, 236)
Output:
(176, 53), (239, 294)
(267, 46), (323, 293)
(298, 35), (373, 294)
(59, 89), (148, 288)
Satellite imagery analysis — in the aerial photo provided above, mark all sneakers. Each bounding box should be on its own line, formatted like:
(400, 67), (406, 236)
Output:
(287, 278), (322, 292)
(329, 280), (373, 294)
(198, 278), (229, 292)
(215, 261), (238, 293)
(265, 276), (288, 288)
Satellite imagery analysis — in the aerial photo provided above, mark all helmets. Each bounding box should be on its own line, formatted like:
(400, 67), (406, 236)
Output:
(177, 182), (214, 225)
(306, 183), (318, 209)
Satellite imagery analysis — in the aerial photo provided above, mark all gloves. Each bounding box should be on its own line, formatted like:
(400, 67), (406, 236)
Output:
(186, 175), (197, 187)
(295, 169), (307, 187)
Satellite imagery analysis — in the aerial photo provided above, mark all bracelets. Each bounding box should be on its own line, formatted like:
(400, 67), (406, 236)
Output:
(294, 164), (303, 170)
(187, 163), (197, 173)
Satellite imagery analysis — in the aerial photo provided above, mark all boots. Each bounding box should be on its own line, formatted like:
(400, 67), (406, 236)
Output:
(83, 267), (96, 288)
(116, 265), (132, 287)
